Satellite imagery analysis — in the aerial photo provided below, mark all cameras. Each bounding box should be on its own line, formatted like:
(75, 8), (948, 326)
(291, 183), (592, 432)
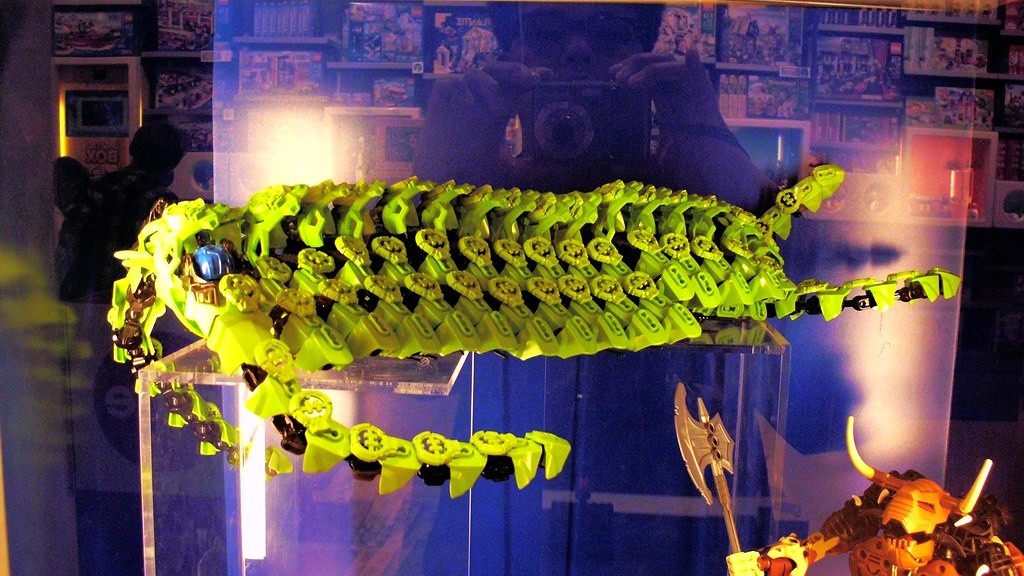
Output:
(532, 81), (652, 161)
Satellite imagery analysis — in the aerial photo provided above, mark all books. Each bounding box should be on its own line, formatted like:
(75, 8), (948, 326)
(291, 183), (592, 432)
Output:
(223, 3), (1024, 222)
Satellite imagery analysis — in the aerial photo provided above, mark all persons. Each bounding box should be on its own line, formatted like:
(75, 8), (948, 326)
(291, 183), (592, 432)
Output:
(404, 6), (858, 575)
(50, 156), (90, 216)
(55, 121), (192, 304)
